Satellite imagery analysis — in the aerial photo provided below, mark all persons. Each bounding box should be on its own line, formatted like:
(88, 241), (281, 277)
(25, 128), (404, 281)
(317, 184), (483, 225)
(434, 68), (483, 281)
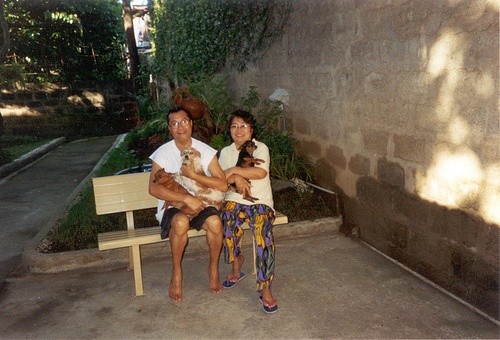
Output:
(148, 107), (228, 303)
(219, 109), (279, 313)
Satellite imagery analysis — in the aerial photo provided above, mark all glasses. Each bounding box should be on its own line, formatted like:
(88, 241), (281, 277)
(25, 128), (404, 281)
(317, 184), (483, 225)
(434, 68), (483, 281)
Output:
(230, 123), (251, 130)
(169, 120), (191, 128)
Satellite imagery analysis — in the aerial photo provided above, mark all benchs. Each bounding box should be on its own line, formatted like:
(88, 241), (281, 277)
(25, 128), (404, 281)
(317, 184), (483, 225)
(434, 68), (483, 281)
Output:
(91, 172), (289, 296)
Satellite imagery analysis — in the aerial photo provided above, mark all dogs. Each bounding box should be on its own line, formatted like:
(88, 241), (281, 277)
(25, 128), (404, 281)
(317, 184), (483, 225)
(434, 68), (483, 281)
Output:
(152, 168), (205, 222)
(227, 140), (265, 203)
(170, 147), (226, 212)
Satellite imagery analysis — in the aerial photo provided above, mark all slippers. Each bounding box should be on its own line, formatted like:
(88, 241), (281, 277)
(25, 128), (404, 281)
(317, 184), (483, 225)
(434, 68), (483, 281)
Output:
(222, 272), (245, 288)
(259, 295), (279, 314)
